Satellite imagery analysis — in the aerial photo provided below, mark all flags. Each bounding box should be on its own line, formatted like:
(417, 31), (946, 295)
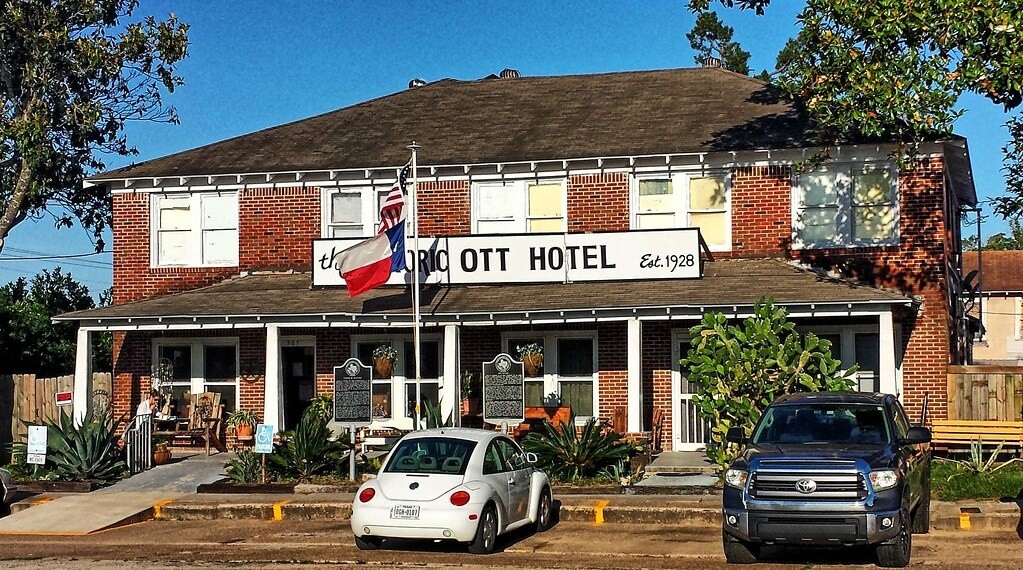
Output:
(378, 157), (412, 234)
(333, 217), (406, 298)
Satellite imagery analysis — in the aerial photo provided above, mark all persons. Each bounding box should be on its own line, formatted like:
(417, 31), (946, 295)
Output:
(136, 390), (160, 431)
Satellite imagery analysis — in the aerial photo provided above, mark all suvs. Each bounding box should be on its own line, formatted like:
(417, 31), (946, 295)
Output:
(722, 391), (938, 568)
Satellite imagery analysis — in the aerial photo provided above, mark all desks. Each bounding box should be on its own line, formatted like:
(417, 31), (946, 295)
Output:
(151, 417), (191, 435)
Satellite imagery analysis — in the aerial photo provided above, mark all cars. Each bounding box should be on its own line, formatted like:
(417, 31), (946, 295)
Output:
(350, 427), (556, 555)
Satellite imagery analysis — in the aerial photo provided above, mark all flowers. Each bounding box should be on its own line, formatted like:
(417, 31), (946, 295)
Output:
(372, 345), (399, 371)
(372, 403), (387, 416)
(515, 343), (543, 368)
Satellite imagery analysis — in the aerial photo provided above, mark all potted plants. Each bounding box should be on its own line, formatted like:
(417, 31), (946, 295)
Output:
(152, 435), (172, 451)
(460, 370), (478, 415)
(228, 410), (260, 436)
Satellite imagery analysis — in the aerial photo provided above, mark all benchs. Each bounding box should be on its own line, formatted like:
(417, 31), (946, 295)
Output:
(359, 435), (403, 455)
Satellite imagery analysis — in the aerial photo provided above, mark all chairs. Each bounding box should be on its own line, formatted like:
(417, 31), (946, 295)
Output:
(442, 457), (463, 473)
(850, 411), (882, 441)
(416, 456), (441, 473)
(396, 456), (421, 474)
(175, 392), (228, 457)
(780, 415), (814, 442)
(600, 406), (663, 454)
(796, 410), (821, 435)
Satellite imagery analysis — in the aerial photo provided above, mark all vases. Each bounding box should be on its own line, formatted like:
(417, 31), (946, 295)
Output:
(521, 354), (541, 378)
(153, 450), (172, 464)
(373, 416), (383, 420)
